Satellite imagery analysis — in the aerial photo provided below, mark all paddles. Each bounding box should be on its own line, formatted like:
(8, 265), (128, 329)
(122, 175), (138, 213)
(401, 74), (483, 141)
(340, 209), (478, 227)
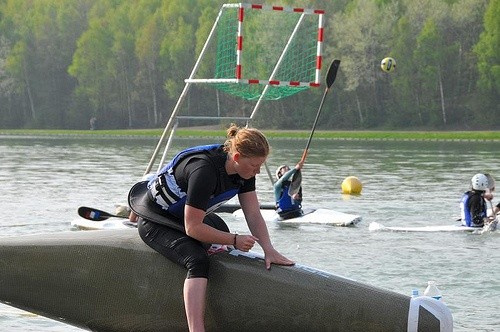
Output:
(75, 206), (137, 221)
(288, 58), (343, 198)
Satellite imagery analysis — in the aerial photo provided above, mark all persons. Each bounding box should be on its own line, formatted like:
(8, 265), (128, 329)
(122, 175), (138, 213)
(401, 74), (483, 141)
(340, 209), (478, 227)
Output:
(274, 161), (304, 221)
(459, 173), (497, 227)
(128, 123), (295, 332)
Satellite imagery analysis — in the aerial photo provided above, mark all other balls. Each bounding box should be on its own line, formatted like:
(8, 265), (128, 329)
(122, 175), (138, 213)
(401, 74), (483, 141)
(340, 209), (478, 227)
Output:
(380, 56), (398, 73)
(341, 175), (362, 195)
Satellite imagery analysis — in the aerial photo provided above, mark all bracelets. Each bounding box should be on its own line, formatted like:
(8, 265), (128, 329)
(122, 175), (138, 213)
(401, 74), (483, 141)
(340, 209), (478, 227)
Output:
(234, 234), (238, 250)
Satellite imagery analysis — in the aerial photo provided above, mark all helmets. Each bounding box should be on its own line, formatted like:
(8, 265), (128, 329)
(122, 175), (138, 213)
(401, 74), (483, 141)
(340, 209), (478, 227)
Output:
(276, 165), (287, 180)
(142, 174), (156, 181)
(471, 173), (488, 193)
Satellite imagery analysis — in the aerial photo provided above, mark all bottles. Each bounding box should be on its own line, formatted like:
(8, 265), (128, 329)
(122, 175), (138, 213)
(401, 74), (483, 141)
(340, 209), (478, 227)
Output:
(423, 281), (443, 302)
(411, 289), (420, 299)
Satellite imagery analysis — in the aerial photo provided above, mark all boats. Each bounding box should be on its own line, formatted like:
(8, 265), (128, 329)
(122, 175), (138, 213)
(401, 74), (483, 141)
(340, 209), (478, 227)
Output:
(370, 213), (500, 236)
(0, 228), (453, 332)
(70, 208), (363, 229)
